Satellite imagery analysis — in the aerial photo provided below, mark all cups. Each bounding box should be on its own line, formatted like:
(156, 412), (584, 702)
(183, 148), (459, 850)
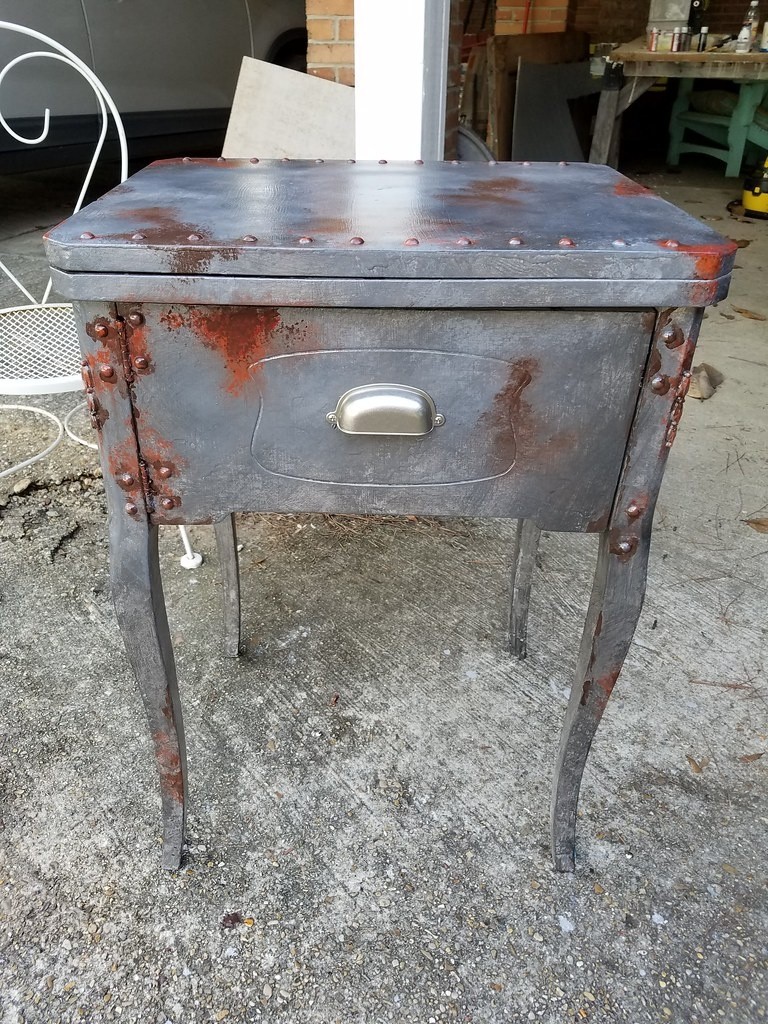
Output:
(759, 22), (768, 52)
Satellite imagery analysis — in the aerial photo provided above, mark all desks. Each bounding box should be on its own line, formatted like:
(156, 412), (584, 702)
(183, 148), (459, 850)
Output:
(44, 158), (742, 874)
(588, 34), (767, 166)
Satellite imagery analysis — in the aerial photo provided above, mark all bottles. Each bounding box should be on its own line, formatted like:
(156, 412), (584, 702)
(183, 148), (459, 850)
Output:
(649, 27), (659, 51)
(698, 26), (708, 52)
(743, 1), (759, 44)
(680, 27), (688, 51)
(735, 23), (751, 53)
(671, 26), (680, 53)
(687, 27), (693, 50)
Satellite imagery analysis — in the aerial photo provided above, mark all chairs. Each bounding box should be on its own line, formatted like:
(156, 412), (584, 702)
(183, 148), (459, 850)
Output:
(1, 21), (200, 572)
